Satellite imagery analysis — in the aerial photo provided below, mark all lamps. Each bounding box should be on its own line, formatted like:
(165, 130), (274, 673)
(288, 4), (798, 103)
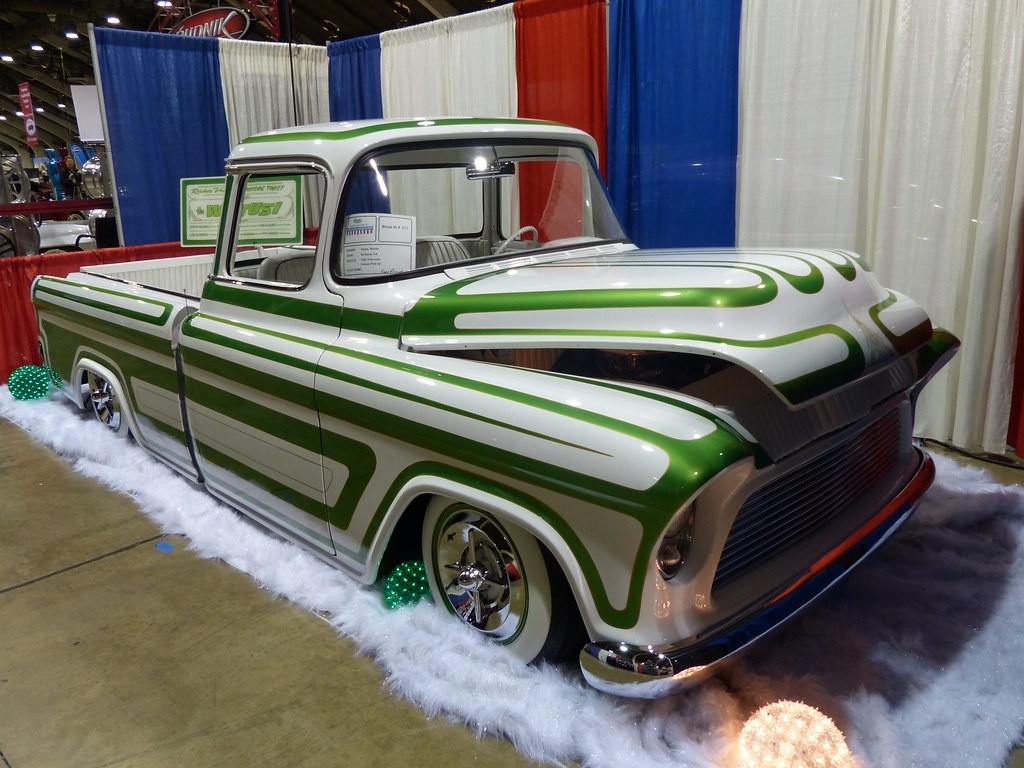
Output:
(28, 39), (44, 52)
(63, 21), (79, 39)
(737, 698), (854, 768)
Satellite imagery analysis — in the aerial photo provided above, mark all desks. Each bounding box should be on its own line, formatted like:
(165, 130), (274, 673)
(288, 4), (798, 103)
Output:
(0, 198), (114, 216)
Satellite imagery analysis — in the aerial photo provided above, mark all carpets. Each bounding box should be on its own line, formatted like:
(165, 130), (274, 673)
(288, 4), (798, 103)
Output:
(0, 380), (1024, 768)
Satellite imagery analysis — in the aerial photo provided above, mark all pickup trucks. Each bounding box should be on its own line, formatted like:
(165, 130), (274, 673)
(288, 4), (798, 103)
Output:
(32, 116), (961, 697)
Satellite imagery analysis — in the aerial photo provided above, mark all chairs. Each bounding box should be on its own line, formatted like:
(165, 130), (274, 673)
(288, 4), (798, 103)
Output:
(75, 217), (119, 251)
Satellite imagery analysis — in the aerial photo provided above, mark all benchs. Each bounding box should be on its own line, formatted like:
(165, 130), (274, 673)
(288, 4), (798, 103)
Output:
(257, 236), (471, 286)
